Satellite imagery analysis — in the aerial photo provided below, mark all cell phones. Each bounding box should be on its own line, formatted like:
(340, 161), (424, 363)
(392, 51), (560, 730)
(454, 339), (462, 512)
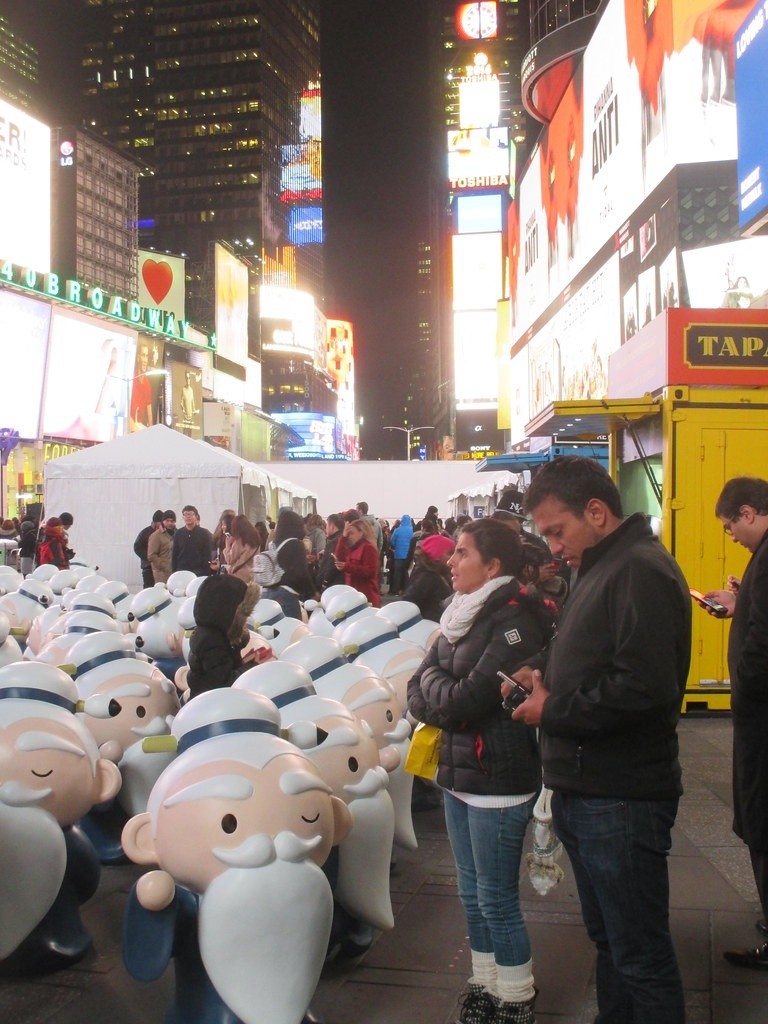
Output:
(689, 588), (728, 614)
(497, 671), (532, 696)
(225, 532), (231, 538)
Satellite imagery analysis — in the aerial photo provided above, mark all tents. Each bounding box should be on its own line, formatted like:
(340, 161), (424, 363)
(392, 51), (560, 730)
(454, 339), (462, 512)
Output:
(43, 420), (318, 597)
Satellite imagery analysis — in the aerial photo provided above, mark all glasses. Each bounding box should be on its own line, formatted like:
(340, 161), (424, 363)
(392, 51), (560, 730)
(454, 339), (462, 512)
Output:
(722, 512), (743, 537)
(184, 512), (195, 517)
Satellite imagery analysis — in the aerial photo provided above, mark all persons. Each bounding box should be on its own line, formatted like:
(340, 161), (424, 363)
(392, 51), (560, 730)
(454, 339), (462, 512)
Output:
(0, 562), (444, 1023)
(0, 511), (77, 579)
(128, 341), (154, 433)
(181, 369), (201, 438)
(132, 455), (768, 1023)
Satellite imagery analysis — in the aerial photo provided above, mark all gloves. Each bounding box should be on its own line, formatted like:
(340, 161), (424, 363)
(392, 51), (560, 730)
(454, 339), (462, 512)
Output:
(66, 549), (76, 560)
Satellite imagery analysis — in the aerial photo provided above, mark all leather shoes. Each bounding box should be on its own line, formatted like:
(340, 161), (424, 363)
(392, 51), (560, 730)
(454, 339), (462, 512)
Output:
(756, 920), (768, 937)
(723, 942), (768, 970)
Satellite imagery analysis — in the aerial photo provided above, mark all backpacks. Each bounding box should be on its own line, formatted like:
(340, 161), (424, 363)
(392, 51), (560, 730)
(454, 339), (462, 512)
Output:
(252, 538), (298, 587)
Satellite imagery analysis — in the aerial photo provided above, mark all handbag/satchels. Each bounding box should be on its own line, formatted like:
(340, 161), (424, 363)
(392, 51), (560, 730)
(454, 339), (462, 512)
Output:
(403, 722), (445, 780)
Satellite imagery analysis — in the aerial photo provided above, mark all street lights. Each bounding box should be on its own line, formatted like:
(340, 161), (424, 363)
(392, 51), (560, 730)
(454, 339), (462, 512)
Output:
(383, 426), (436, 460)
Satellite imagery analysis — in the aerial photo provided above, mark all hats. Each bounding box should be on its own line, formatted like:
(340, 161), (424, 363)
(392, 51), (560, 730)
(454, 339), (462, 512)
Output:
(47, 517), (61, 527)
(59, 512), (73, 525)
(526, 784), (563, 896)
(22, 521), (34, 532)
(491, 490), (528, 520)
(162, 510), (176, 521)
(153, 510), (164, 521)
(428, 506), (438, 514)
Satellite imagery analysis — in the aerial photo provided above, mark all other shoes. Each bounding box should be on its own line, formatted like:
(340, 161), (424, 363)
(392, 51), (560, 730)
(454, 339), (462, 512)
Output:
(388, 590), (399, 596)
(455, 977), (539, 1024)
